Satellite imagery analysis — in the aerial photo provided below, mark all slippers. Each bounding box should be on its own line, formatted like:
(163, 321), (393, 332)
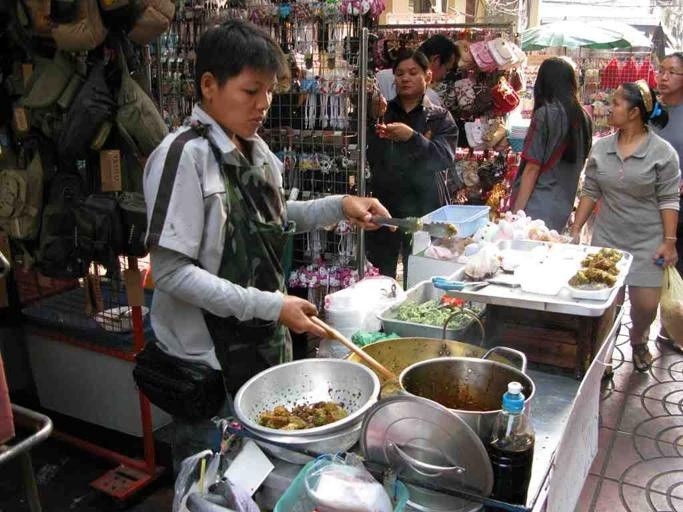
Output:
(631, 342), (652, 371)
(656, 333), (683, 354)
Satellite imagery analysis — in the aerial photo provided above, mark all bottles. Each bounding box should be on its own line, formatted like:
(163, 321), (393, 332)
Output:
(486, 381), (536, 508)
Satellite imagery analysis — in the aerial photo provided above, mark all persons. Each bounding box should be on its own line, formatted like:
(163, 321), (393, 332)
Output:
(646, 51), (682, 354)
(137, 16), (400, 479)
(353, 47), (462, 293)
(509, 55), (594, 234)
(371, 34), (462, 112)
(567, 78), (681, 373)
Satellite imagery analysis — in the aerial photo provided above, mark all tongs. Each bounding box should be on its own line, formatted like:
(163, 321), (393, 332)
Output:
(353, 214), (458, 241)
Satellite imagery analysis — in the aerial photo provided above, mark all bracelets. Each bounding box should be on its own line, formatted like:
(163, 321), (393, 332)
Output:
(663, 234), (678, 242)
(568, 222), (583, 232)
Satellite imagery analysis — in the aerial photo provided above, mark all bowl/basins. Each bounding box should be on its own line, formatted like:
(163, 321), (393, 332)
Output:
(230, 357), (382, 468)
(92, 303), (149, 333)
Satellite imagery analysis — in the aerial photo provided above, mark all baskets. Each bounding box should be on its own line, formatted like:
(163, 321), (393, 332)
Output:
(273, 460), (409, 512)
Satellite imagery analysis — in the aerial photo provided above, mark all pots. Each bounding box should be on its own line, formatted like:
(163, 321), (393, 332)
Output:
(344, 310), (537, 448)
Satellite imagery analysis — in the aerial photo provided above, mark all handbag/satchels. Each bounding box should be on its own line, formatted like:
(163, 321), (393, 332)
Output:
(0, 1), (175, 279)
(344, 30), (432, 70)
(451, 38), (527, 72)
(132, 341), (224, 424)
(433, 76), (520, 206)
